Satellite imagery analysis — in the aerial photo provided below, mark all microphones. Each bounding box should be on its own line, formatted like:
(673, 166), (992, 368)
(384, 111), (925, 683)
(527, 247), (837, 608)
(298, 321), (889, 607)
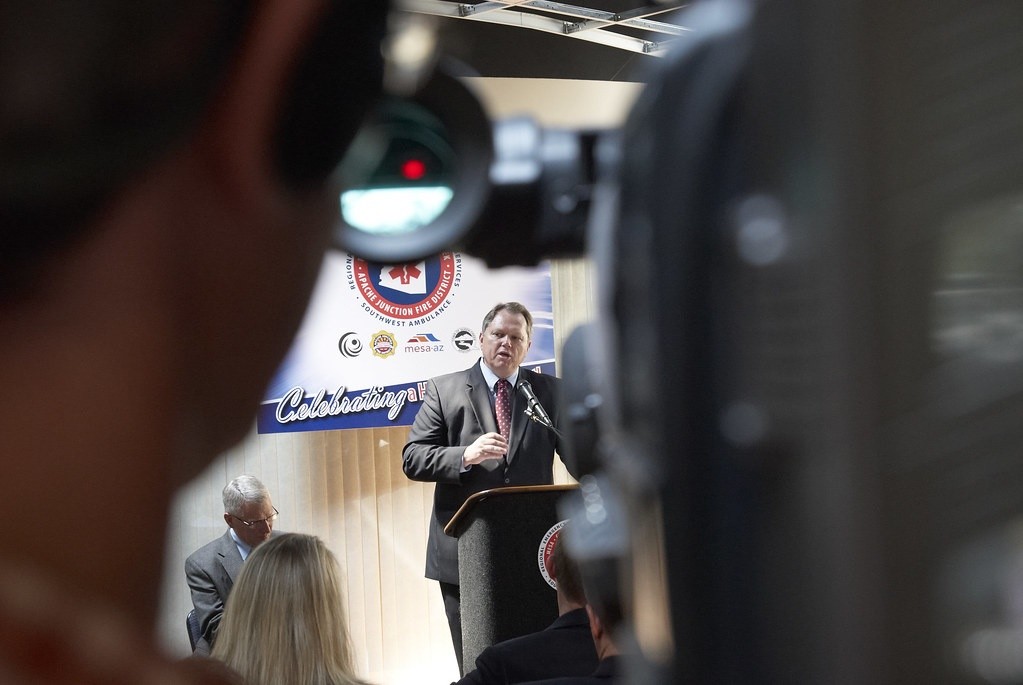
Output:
(516, 379), (553, 430)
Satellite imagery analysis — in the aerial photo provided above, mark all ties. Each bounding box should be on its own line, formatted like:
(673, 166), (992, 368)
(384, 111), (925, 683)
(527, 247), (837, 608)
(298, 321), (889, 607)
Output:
(495, 377), (512, 455)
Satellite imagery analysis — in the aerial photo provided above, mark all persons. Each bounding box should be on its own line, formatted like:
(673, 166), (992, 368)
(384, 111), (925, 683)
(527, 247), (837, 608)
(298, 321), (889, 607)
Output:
(211, 530), (358, 685)
(0, 0), (391, 684)
(184, 475), (279, 657)
(444, 514), (648, 685)
(403, 302), (566, 672)
(591, 0), (1023, 685)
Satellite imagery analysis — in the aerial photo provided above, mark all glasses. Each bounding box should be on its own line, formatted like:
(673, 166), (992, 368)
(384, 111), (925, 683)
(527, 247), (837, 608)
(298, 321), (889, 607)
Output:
(229, 506), (279, 529)
